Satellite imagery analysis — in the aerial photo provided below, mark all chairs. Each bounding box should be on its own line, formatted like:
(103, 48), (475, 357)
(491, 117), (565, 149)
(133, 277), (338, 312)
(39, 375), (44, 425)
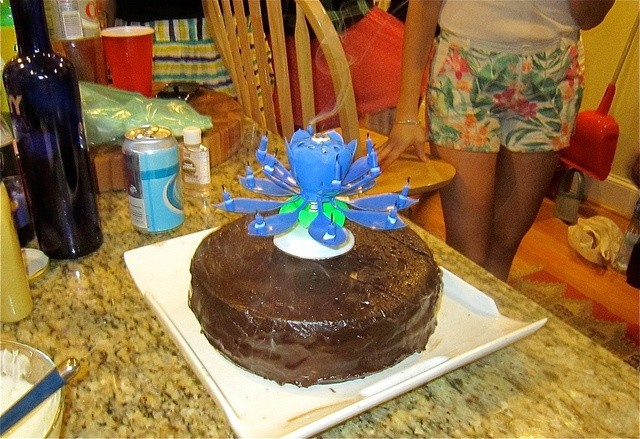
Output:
(199, 0), (456, 244)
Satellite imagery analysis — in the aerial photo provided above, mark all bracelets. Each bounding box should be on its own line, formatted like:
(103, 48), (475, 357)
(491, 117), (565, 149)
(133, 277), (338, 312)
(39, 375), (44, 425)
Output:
(391, 120), (423, 127)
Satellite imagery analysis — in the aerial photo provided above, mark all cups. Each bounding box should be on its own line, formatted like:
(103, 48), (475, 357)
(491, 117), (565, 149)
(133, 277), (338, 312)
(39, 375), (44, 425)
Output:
(96, 21), (160, 100)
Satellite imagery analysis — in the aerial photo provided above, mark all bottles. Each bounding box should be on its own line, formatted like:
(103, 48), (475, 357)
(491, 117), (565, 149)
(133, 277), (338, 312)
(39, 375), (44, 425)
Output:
(49, 0), (109, 86)
(1, 0), (103, 258)
(1, 181), (33, 323)
(177, 126), (211, 233)
(122, 124), (185, 237)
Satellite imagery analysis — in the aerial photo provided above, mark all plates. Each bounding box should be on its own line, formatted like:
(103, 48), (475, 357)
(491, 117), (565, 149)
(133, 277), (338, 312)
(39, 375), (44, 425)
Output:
(120, 213), (558, 433)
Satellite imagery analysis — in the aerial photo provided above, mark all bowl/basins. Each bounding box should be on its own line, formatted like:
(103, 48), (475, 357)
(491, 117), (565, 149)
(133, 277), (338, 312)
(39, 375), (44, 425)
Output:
(1, 332), (94, 436)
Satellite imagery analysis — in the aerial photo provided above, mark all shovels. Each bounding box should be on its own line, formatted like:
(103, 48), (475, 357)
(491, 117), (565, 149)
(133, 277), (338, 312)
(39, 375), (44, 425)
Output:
(558, 10), (640, 182)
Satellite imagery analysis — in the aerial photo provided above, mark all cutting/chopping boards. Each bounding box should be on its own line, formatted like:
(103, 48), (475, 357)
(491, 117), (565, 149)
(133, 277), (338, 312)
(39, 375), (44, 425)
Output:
(66, 72), (252, 197)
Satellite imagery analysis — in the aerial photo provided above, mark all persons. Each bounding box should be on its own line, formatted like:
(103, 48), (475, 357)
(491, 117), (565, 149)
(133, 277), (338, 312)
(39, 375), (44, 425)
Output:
(260, 0), (437, 132)
(374, 0), (616, 282)
(111, 0), (275, 126)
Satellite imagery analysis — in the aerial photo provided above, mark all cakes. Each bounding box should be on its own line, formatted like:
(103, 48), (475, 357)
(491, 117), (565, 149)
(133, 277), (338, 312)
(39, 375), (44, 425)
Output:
(187, 202), (442, 389)
